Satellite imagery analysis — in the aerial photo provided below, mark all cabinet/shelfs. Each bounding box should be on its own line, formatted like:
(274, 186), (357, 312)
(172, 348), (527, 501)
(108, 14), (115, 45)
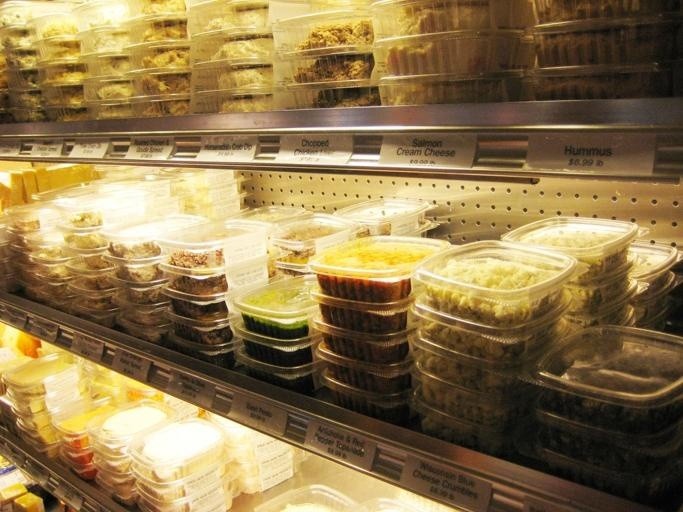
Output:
(0, 96), (683, 512)
